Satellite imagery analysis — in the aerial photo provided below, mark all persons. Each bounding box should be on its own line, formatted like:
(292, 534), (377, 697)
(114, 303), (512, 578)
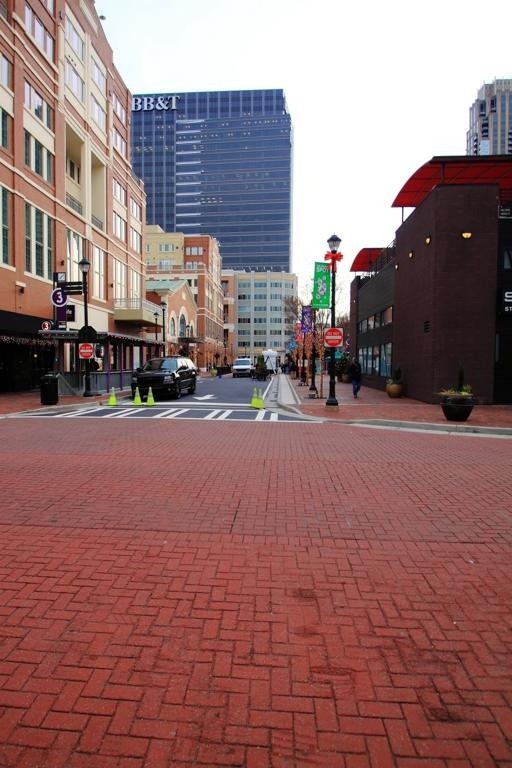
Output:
(348, 357), (362, 398)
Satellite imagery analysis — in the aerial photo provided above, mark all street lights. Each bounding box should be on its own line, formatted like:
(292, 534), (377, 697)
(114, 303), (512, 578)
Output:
(76, 255), (106, 398)
(323, 233), (342, 407)
(153, 311), (160, 357)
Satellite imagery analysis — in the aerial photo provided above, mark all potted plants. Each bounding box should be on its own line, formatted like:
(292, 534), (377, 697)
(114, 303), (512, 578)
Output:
(342, 365), (350, 382)
(385, 362), (404, 397)
(437, 384), (475, 422)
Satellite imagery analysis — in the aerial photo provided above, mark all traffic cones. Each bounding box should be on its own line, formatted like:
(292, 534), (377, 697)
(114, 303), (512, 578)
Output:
(144, 386), (158, 407)
(106, 385), (120, 407)
(257, 387), (266, 409)
(131, 385), (145, 407)
(250, 385), (260, 407)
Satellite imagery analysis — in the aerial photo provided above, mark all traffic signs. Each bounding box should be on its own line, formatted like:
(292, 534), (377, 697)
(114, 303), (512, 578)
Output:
(322, 326), (345, 349)
(78, 342), (98, 362)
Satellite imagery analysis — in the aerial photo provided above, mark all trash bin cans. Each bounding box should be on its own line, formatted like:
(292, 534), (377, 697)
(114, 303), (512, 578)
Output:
(39, 372), (59, 405)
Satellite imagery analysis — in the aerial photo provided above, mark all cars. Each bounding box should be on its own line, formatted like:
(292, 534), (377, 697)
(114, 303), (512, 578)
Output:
(231, 357), (257, 379)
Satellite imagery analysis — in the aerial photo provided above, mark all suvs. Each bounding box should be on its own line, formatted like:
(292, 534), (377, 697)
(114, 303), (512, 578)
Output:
(130, 355), (199, 401)
